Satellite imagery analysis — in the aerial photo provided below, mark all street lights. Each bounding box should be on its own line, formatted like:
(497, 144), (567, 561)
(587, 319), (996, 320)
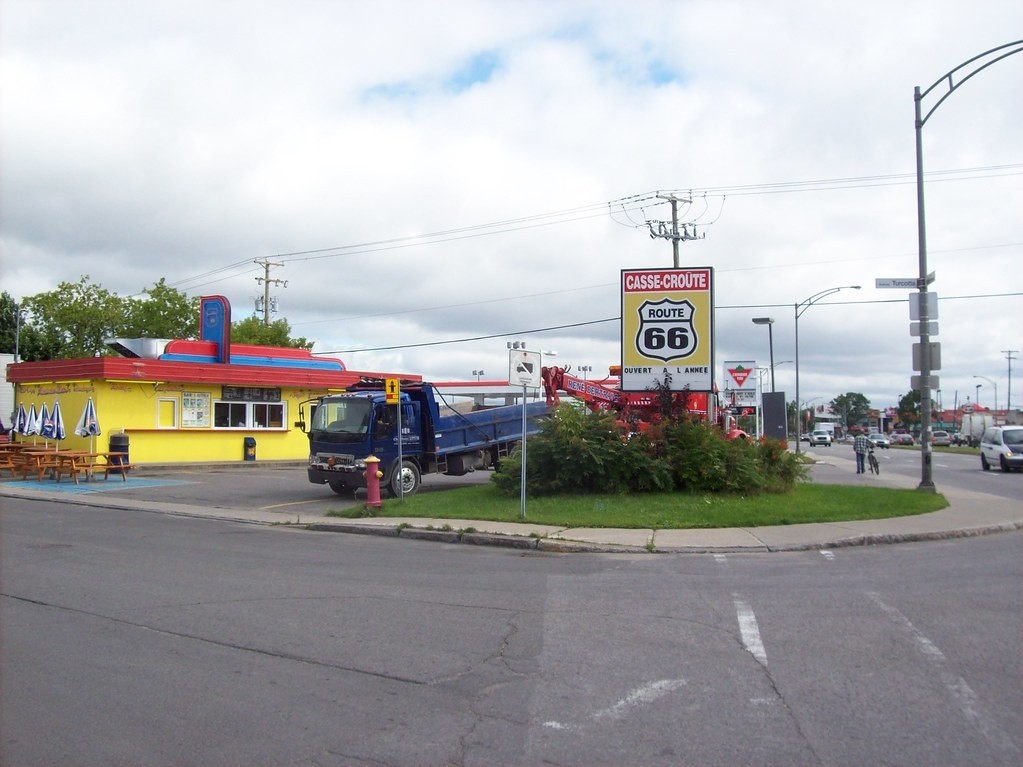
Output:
(751, 317), (776, 393)
(976, 384), (982, 414)
(973, 375), (998, 426)
(794, 286), (862, 456)
(760, 359), (794, 444)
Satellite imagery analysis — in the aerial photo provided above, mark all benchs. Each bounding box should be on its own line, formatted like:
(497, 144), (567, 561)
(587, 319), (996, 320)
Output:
(0, 460), (9, 462)
(18, 460), (72, 464)
(48, 464), (106, 467)
(11, 455), (24, 458)
(0, 464), (15, 467)
(93, 465), (129, 468)
(13, 458), (26, 461)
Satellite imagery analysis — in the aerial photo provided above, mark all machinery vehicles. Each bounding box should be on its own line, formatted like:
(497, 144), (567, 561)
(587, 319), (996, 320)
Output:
(541, 364), (752, 452)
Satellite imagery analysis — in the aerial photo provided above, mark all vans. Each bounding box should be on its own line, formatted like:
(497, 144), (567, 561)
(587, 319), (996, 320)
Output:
(978, 423), (1023, 474)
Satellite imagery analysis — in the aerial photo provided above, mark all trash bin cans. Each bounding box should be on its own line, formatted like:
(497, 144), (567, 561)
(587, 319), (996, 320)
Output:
(107, 433), (130, 474)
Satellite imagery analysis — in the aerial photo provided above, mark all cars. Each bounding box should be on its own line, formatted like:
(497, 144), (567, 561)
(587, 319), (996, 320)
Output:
(809, 429), (832, 448)
(837, 434), (855, 444)
(918, 430), (952, 448)
(800, 433), (810, 442)
(867, 432), (915, 449)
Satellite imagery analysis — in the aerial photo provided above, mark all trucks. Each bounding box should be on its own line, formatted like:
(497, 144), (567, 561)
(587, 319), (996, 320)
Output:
(294, 375), (557, 500)
(953, 413), (997, 448)
(814, 423), (835, 442)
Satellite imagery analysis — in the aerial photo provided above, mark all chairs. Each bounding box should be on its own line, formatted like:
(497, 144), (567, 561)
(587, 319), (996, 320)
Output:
(384, 407), (392, 423)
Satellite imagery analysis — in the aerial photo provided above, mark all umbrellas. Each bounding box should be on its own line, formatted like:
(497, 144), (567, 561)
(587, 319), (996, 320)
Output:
(12, 402), (27, 445)
(22, 403), (38, 447)
(34, 401), (53, 449)
(75, 396), (102, 478)
(50, 400), (66, 475)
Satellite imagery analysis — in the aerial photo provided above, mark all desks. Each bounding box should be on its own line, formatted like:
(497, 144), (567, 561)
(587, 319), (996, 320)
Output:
(0, 441), (129, 485)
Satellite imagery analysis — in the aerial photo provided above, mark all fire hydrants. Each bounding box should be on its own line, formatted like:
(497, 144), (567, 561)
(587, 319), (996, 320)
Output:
(362, 454), (383, 508)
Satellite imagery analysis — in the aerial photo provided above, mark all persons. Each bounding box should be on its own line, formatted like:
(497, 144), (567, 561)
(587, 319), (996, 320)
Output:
(954, 429), (959, 443)
(853, 429), (876, 473)
